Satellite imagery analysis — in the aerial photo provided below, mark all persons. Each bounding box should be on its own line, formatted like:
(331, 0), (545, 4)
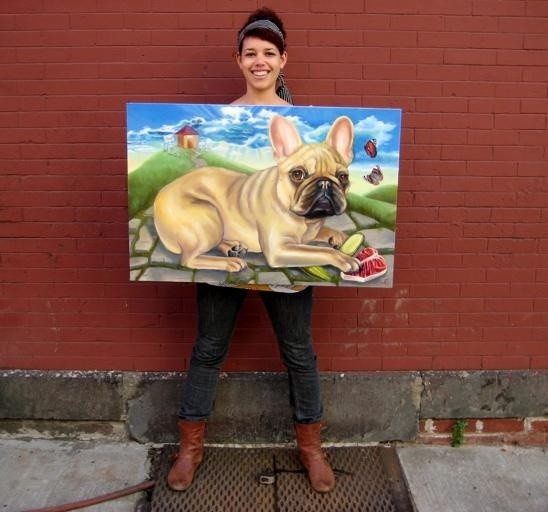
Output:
(166, 6), (337, 493)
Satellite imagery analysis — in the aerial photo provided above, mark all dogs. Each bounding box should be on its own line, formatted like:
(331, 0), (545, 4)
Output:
(153, 113), (362, 274)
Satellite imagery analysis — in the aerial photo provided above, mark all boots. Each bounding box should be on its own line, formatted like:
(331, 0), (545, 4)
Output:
(167, 420), (206, 491)
(293, 420), (335, 493)
